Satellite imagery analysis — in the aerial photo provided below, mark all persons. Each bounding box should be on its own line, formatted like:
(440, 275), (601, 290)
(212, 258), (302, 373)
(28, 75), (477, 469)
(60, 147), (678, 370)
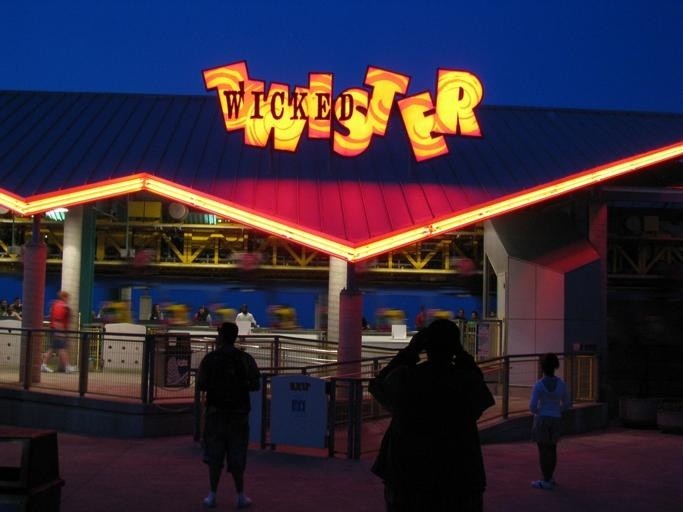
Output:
(148, 304), (165, 321)
(453, 309), (480, 355)
(416, 310), (425, 328)
(40, 290), (80, 373)
(0, 298), (23, 321)
(529, 352), (570, 492)
(196, 304), (213, 327)
(193, 322), (260, 507)
(235, 304), (259, 336)
(369, 318), (496, 512)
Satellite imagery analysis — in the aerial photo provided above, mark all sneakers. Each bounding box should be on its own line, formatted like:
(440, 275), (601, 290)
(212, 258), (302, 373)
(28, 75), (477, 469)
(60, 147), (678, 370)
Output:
(239, 497), (252, 507)
(41, 365), (54, 373)
(203, 496), (217, 506)
(531, 481), (551, 488)
